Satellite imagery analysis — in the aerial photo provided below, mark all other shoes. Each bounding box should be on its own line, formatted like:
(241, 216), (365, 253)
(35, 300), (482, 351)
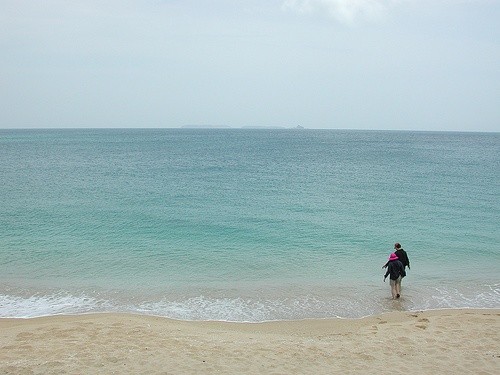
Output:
(397, 294), (400, 298)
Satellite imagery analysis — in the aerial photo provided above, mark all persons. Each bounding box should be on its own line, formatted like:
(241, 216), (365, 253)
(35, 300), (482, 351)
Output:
(383, 252), (406, 301)
(381, 242), (411, 294)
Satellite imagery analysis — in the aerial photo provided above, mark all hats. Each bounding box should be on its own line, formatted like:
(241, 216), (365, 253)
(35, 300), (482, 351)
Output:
(388, 252), (399, 260)
(394, 242), (399, 249)
